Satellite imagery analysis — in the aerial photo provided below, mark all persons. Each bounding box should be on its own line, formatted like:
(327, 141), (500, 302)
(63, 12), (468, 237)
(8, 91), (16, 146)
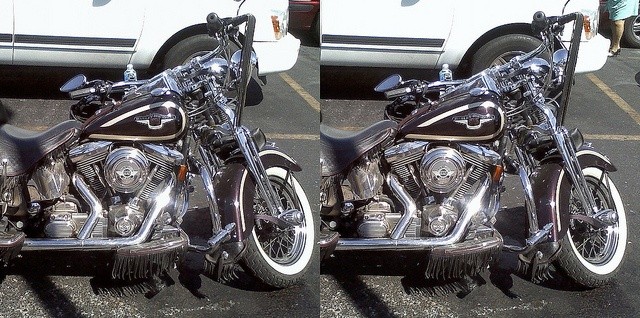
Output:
(607, 0), (639, 57)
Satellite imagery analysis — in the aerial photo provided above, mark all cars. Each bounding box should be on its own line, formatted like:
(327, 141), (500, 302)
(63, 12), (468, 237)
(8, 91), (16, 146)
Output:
(320, 0), (613, 91)
(598, 0), (640, 50)
(288, 0), (319, 48)
(2, 0), (303, 86)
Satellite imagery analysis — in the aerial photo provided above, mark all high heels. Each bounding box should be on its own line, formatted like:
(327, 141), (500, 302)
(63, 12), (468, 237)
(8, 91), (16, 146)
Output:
(608, 49), (621, 57)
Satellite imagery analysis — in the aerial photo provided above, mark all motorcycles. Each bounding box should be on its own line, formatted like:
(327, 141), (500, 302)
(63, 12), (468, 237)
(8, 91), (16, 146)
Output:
(320, 0), (630, 291)
(1, 0), (319, 289)
